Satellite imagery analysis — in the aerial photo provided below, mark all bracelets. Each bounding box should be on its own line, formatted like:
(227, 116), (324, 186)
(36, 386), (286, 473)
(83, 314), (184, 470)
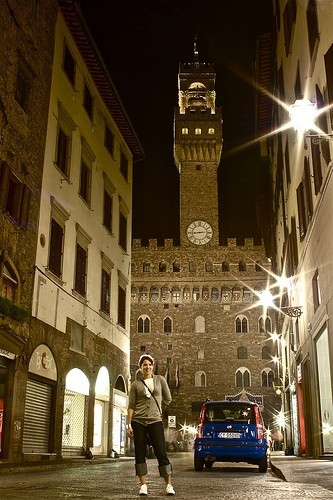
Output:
(126, 424), (131, 429)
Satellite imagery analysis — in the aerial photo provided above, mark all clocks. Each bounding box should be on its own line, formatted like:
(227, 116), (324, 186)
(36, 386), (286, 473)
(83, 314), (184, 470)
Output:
(186, 219), (215, 246)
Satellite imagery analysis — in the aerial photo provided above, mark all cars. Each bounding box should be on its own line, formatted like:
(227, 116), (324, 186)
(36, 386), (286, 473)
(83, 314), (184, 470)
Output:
(193, 400), (269, 474)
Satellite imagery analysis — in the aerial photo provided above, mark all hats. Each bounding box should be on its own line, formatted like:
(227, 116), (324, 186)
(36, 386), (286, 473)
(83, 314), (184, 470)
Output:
(138, 354), (155, 369)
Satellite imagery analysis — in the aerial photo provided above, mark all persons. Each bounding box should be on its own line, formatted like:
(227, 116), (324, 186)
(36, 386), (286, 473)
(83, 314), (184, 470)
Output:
(213, 410), (226, 420)
(176, 430), (183, 452)
(184, 431), (190, 452)
(125, 354), (175, 496)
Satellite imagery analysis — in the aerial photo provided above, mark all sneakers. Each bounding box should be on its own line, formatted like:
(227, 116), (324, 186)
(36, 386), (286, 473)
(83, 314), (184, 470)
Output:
(165, 484), (176, 495)
(139, 485), (148, 495)
